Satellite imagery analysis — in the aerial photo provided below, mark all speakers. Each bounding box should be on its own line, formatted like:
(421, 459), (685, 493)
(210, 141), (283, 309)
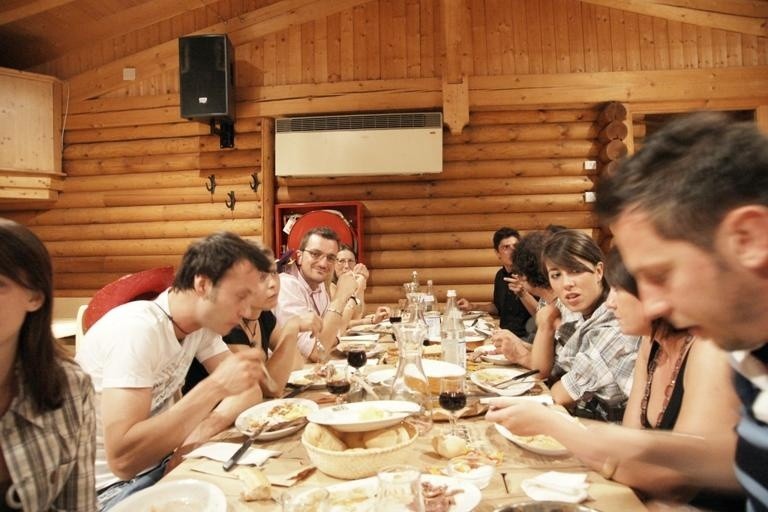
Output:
(178, 33), (238, 125)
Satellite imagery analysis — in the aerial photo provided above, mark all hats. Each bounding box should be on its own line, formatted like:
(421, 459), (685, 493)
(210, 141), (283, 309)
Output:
(79, 262), (176, 337)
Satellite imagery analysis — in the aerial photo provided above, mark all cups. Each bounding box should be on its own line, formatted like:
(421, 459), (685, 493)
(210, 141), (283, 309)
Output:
(282, 484), (331, 512)
(390, 307), (403, 322)
(326, 364), (347, 405)
(376, 467), (424, 511)
(347, 345), (367, 381)
(439, 377), (469, 440)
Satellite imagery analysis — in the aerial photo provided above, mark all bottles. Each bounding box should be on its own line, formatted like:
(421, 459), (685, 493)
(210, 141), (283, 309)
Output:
(442, 290), (467, 372)
(425, 282), (437, 316)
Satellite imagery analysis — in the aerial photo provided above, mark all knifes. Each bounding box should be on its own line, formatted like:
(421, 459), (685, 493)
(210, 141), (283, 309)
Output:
(281, 381), (316, 401)
(222, 421), (271, 471)
(490, 369), (541, 391)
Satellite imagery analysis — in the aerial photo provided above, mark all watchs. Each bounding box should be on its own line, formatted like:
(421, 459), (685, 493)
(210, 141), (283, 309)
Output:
(348, 296), (358, 304)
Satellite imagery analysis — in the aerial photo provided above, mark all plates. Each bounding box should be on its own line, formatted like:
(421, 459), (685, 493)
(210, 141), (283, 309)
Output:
(111, 478), (228, 512)
(494, 419), (571, 456)
(305, 399), (420, 434)
(235, 397), (319, 441)
(281, 470), (483, 512)
(474, 344), (517, 365)
(428, 319), (485, 342)
(286, 358), (354, 388)
(349, 323), (391, 333)
(339, 334), (385, 357)
(470, 367), (536, 398)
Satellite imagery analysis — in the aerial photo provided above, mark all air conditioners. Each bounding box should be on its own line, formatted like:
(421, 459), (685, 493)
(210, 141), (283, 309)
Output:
(274, 111), (445, 176)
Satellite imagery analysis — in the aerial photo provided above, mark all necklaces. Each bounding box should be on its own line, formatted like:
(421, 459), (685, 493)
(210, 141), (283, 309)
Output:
(639, 335), (692, 427)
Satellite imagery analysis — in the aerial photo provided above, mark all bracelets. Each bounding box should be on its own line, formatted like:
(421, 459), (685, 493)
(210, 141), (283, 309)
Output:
(325, 307), (344, 319)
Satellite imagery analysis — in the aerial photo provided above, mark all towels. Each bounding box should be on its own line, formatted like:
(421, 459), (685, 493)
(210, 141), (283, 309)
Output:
(519, 470), (598, 506)
(179, 440), (283, 468)
(478, 392), (557, 408)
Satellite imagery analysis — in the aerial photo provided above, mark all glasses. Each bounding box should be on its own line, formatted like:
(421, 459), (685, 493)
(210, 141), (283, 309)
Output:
(303, 249), (339, 265)
(337, 259), (356, 264)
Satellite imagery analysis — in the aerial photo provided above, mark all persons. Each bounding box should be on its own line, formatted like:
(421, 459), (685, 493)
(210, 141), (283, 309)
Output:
(492, 232), (561, 368)
(485, 245), (746, 496)
(181, 246), (321, 398)
(531, 231), (639, 404)
(77, 232), (264, 506)
(0, 219), (95, 512)
(455, 226), (530, 340)
(334, 247), (369, 320)
(274, 228), (358, 362)
(597, 111), (768, 512)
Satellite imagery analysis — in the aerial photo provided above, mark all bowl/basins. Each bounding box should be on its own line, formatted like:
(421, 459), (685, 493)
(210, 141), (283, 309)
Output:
(494, 500), (603, 512)
(404, 358), (467, 395)
(461, 336), (486, 351)
